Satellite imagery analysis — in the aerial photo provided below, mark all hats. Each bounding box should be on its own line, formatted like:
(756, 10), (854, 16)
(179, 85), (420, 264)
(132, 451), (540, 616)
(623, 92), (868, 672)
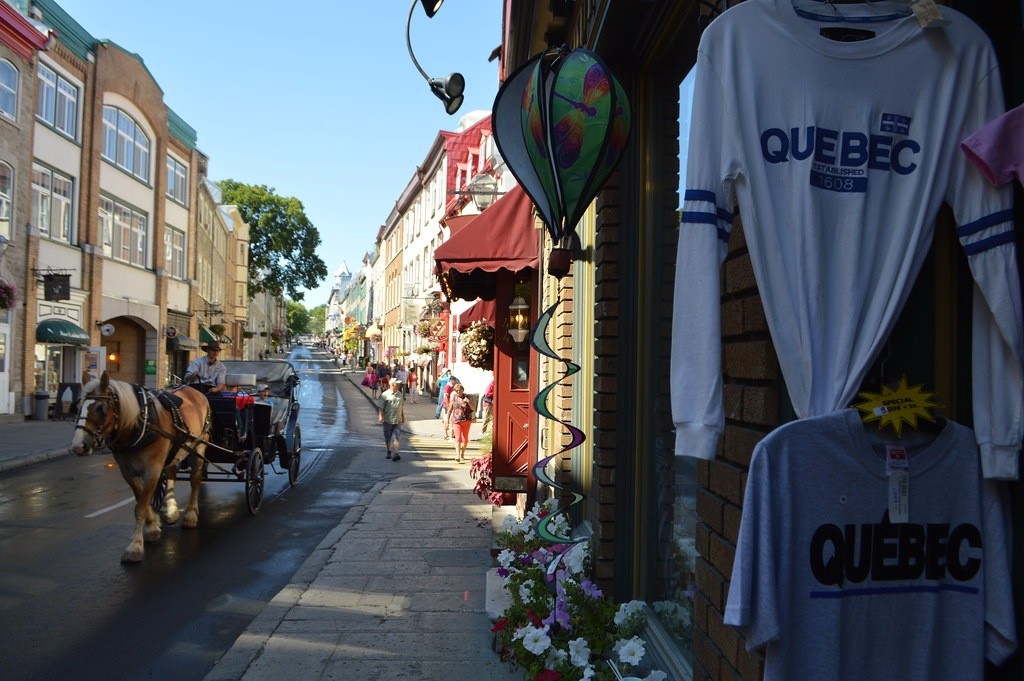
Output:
(440, 367), (451, 378)
(388, 377), (403, 385)
(449, 375), (457, 380)
(256, 384), (271, 393)
(201, 340), (222, 353)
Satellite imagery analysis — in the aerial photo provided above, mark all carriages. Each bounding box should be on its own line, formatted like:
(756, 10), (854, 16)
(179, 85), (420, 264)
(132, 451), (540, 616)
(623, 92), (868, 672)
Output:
(71, 360), (303, 565)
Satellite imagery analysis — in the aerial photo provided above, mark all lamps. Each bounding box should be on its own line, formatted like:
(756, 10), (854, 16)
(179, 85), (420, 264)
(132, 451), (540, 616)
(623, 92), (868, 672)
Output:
(466, 172), (498, 212)
(508, 293), (530, 344)
(406, 0), (465, 116)
(421, 0), (444, 18)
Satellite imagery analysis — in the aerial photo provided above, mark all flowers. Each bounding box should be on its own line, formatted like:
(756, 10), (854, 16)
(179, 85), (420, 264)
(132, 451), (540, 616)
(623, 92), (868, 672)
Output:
(414, 319), (431, 339)
(0, 281), (20, 312)
(460, 318), (492, 369)
(469, 452), (504, 508)
(488, 496), (667, 681)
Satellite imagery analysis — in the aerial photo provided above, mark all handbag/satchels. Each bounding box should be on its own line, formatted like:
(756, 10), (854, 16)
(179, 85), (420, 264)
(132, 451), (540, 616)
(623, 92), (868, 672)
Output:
(481, 394), (493, 403)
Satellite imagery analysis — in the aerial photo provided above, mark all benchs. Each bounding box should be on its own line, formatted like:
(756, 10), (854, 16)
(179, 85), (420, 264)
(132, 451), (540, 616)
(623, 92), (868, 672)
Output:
(252, 395), (289, 434)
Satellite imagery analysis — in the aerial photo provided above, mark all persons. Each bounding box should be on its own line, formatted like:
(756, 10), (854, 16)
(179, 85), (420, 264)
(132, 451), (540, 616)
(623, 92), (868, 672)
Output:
(446, 383), (474, 462)
(443, 376), (456, 414)
(379, 361), (390, 391)
(351, 356), (356, 373)
(183, 341), (227, 396)
(361, 362), (373, 387)
(321, 343), (326, 348)
(255, 384), (274, 409)
(265, 349), (269, 360)
(342, 356), (347, 365)
(395, 365), (408, 401)
(434, 368), (459, 419)
(371, 369), (378, 398)
(408, 368), (419, 404)
(259, 350), (264, 360)
(481, 381), (494, 434)
(377, 378), (405, 461)
(394, 359), (400, 372)
(222, 384), (249, 396)
(363, 356), (368, 370)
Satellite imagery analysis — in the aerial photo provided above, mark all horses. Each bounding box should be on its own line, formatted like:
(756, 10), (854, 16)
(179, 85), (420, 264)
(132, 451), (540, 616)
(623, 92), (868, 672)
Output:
(71, 368), (211, 564)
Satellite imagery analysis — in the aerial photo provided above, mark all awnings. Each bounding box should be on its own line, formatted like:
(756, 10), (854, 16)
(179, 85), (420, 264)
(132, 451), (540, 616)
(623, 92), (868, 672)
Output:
(199, 325), (232, 343)
(458, 299), (496, 334)
(432, 183), (544, 301)
(167, 335), (197, 351)
(36, 320), (89, 345)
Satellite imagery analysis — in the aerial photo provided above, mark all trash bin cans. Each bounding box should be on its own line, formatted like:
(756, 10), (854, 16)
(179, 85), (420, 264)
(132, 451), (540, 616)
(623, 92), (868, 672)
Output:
(35, 391), (49, 420)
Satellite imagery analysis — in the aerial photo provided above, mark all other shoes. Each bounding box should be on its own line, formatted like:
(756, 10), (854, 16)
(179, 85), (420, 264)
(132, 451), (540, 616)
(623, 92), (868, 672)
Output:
(434, 415), (439, 419)
(392, 453), (401, 461)
(455, 457), (460, 462)
(410, 401), (418, 404)
(460, 457), (465, 464)
(385, 451), (392, 459)
(404, 397), (406, 401)
(444, 435), (450, 440)
(451, 434), (455, 438)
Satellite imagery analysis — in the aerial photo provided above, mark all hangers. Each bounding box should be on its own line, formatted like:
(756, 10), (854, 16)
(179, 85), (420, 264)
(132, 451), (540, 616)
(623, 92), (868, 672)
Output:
(795, 0), (911, 17)
(874, 356), (946, 433)
